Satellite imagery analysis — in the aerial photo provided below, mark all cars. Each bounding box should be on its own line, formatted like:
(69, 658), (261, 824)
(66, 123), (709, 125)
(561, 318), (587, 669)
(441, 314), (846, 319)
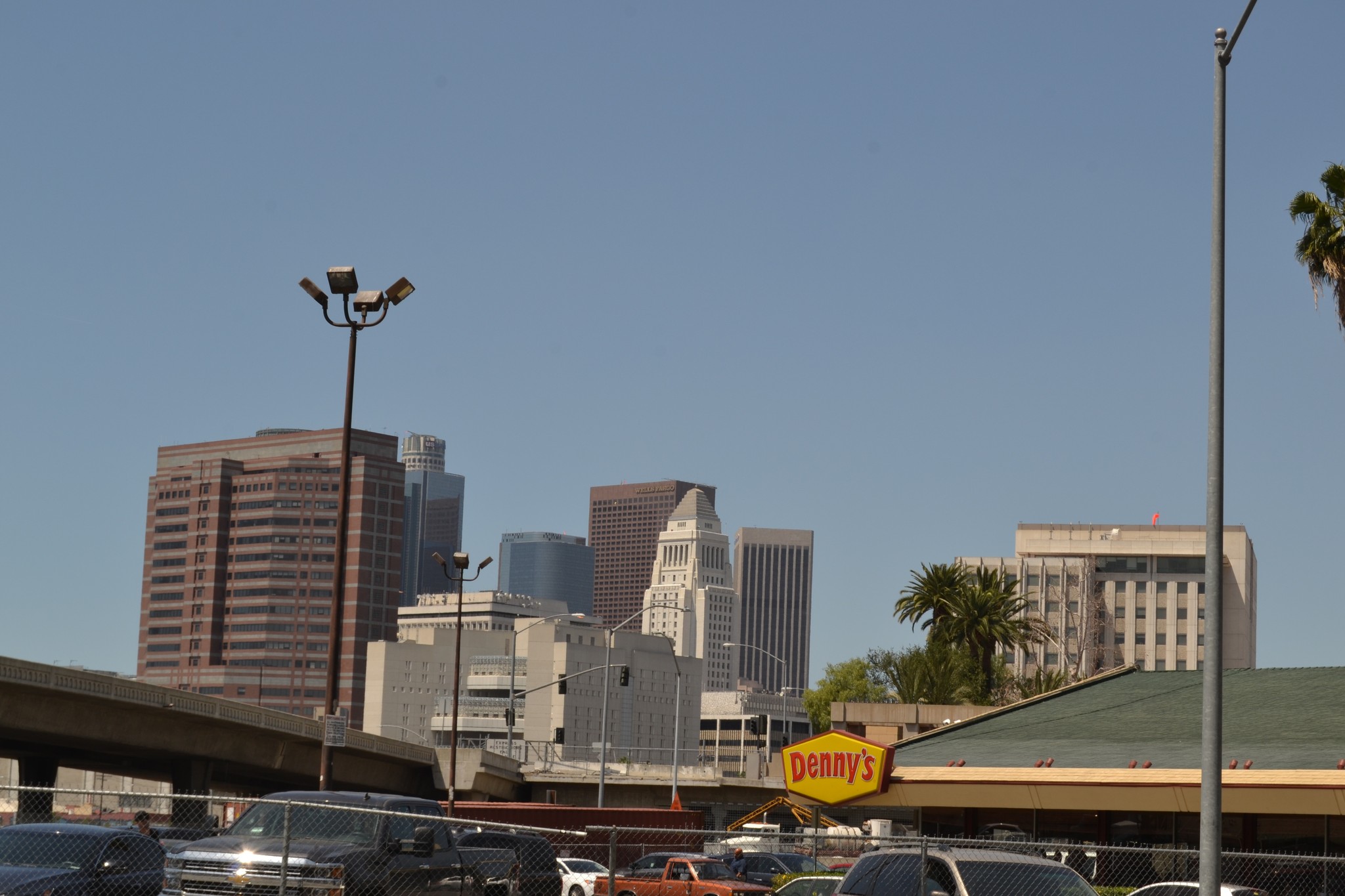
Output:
(556, 857), (624, 896)
(776, 877), (843, 896)
(0, 822), (169, 896)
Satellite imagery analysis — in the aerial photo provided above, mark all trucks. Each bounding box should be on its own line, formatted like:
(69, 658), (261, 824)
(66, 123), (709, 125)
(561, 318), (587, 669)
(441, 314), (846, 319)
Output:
(720, 819), (893, 857)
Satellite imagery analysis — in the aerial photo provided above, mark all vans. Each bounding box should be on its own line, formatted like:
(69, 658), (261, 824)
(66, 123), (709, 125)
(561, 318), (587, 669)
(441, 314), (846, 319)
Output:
(448, 825), (563, 896)
(1124, 881), (1270, 896)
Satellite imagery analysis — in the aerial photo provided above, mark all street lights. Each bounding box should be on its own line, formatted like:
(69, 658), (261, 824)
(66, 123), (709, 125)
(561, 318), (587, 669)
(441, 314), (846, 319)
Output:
(598, 605), (692, 808)
(300, 267), (415, 791)
(506, 613), (585, 758)
(652, 631), (682, 806)
(432, 552), (493, 817)
(723, 643), (786, 747)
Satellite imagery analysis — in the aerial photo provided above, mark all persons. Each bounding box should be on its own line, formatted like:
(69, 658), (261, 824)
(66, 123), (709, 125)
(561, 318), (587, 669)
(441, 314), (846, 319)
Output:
(134, 810), (159, 844)
(1064, 849), (1071, 867)
(730, 848), (748, 882)
(1053, 849), (1062, 863)
(1039, 847), (1047, 859)
(697, 864), (718, 880)
(859, 839), (871, 854)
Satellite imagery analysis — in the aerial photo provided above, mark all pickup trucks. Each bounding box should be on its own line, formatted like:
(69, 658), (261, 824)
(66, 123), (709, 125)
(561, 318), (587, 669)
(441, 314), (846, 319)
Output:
(593, 857), (778, 896)
(157, 791), (519, 896)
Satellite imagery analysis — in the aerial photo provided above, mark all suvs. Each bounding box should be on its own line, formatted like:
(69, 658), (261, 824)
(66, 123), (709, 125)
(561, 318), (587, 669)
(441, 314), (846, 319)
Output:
(616, 852), (708, 879)
(707, 852), (835, 888)
(830, 844), (1101, 896)
